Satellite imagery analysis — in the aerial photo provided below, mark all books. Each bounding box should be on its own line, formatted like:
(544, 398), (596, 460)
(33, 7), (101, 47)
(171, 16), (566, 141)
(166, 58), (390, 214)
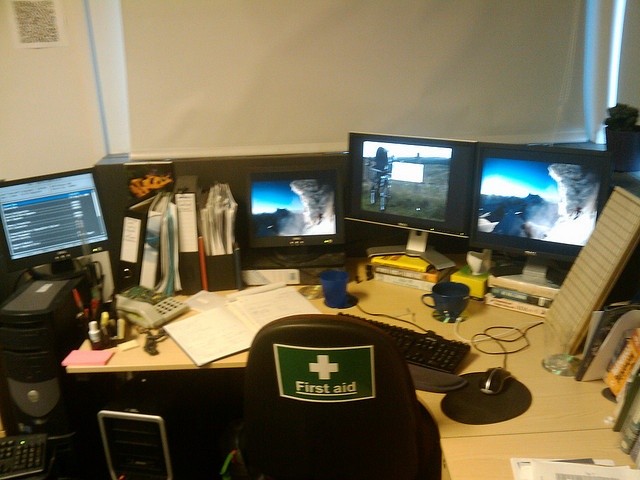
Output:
(370, 252), (434, 272)
(574, 300), (640, 470)
(373, 269), (459, 291)
(371, 264), (452, 282)
(155, 201), (183, 293)
(491, 285), (554, 309)
(485, 292), (550, 318)
(161, 286), (324, 367)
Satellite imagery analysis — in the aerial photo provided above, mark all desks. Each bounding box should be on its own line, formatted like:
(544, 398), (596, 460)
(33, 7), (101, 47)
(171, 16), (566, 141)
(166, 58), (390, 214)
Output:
(66, 256), (640, 436)
(437, 428), (640, 480)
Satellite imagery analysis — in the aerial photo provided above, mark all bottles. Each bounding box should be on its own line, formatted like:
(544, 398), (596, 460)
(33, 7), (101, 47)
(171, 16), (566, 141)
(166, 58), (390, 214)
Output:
(88, 320), (102, 342)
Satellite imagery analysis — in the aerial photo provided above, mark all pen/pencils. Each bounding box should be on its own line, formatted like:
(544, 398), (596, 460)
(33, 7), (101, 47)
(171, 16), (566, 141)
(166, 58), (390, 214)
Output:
(72, 288), (84, 311)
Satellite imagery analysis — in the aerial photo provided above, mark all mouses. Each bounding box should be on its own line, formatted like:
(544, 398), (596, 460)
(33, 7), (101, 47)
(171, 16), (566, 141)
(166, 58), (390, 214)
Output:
(479, 366), (511, 394)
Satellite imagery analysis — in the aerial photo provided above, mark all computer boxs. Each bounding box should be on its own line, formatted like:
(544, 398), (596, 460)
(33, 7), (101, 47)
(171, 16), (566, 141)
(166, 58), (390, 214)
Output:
(1, 275), (85, 441)
(96, 371), (174, 478)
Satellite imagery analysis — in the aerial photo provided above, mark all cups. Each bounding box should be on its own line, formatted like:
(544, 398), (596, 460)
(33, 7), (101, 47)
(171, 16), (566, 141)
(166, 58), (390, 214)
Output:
(421, 281), (471, 318)
(319, 270), (348, 307)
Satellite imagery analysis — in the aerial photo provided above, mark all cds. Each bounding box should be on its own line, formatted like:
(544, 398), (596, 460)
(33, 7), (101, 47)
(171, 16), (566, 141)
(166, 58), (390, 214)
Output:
(542, 354), (583, 378)
(432, 308), (460, 323)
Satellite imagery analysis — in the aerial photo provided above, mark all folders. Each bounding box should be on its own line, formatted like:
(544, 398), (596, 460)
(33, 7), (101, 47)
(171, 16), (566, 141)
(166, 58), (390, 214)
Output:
(117, 195), (157, 289)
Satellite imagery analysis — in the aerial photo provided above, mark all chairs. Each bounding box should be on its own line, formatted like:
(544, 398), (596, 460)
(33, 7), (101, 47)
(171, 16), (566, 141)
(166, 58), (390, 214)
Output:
(235, 314), (442, 480)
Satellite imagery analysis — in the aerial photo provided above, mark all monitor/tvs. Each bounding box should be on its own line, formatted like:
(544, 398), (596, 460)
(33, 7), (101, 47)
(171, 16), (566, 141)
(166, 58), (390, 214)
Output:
(0, 168), (114, 281)
(469, 141), (612, 286)
(245, 168), (344, 269)
(345, 130), (478, 271)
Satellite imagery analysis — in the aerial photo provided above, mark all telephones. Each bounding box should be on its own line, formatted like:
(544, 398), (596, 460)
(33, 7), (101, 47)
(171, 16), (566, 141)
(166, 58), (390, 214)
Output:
(111, 284), (189, 355)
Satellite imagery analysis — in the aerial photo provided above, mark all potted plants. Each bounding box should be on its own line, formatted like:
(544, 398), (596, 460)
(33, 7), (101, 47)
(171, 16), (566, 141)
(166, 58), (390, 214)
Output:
(604, 103), (640, 173)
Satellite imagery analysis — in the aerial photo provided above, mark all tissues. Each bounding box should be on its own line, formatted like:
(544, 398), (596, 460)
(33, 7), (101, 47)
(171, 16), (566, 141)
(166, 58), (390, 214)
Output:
(449, 249), (496, 298)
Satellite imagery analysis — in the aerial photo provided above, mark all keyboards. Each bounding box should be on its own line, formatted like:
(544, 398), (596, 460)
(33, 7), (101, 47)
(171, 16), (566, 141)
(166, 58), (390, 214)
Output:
(336, 311), (470, 374)
(0, 433), (49, 480)
